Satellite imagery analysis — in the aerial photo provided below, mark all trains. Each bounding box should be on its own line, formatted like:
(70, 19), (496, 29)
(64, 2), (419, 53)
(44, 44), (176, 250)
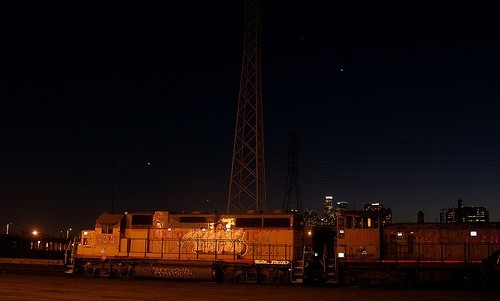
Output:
(68, 210), (499, 285)
